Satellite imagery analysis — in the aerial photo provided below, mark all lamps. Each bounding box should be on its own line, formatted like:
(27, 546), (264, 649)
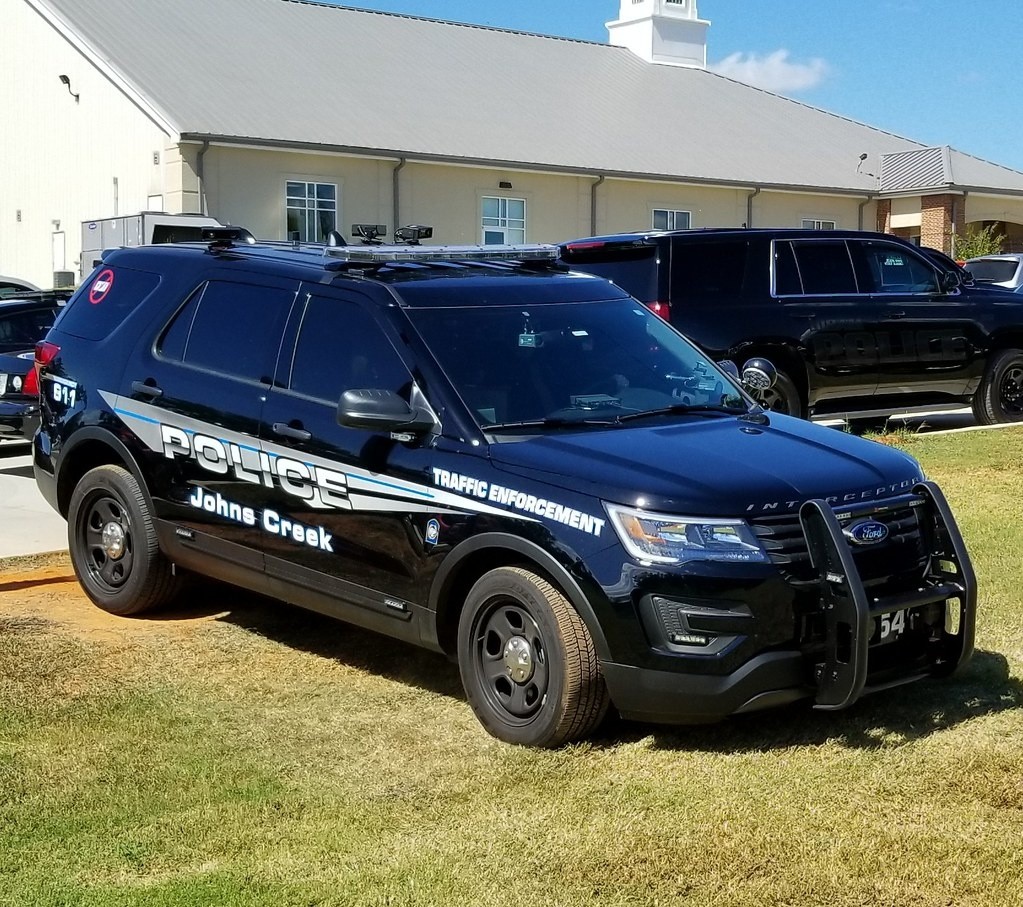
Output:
(59, 74), (79, 103)
(857, 153), (867, 173)
(499, 182), (513, 188)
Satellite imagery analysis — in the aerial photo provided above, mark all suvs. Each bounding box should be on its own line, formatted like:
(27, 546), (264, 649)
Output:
(550, 228), (1023, 435)
(29, 222), (982, 746)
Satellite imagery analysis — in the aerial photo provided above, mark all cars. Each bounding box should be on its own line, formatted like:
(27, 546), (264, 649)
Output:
(0, 276), (77, 445)
(959, 253), (1023, 296)
(877, 247), (975, 295)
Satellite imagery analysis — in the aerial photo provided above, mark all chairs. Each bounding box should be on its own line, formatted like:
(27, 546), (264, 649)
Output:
(508, 331), (591, 419)
